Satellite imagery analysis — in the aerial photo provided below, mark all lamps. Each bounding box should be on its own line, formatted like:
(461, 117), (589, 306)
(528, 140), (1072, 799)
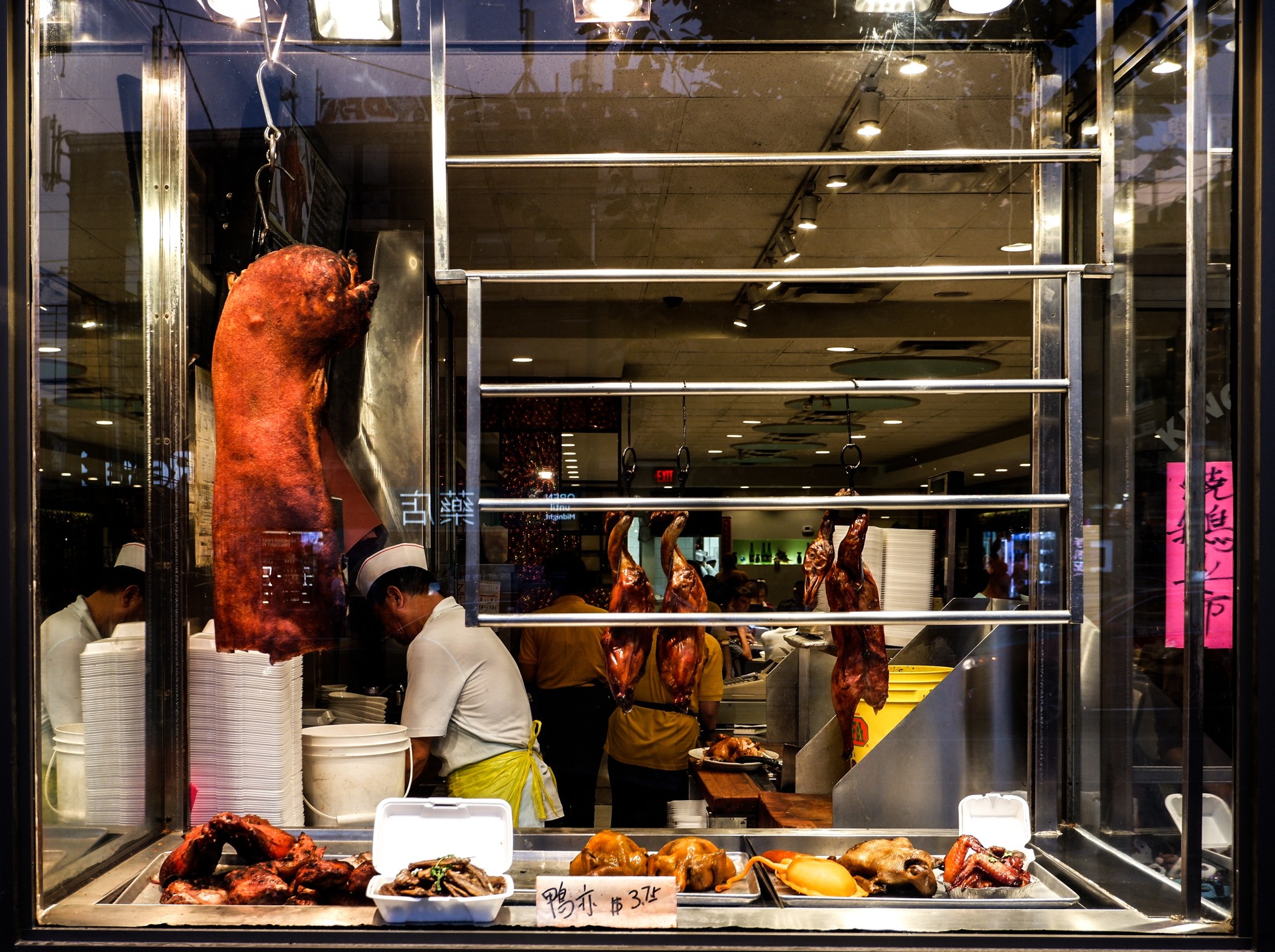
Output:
(935, 0), (1023, 21)
(845, 0), (946, 23)
(197, 0), (285, 22)
(826, 134), (847, 187)
(308, 0), (401, 47)
(1152, 5), (1182, 74)
(573, 0), (651, 23)
(858, 77), (883, 135)
(734, 182), (820, 327)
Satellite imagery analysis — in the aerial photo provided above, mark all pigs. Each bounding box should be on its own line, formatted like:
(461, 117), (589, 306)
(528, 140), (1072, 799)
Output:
(209, 239), (381, 668)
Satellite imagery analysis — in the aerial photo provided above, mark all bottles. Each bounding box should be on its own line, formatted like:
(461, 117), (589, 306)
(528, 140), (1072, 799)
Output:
(761, 543), (767, 564)
(755, 554), (761, 565)
(797, 551), (803, 564)
(749, 542), (754, 564)
(767, 542), (772, 565)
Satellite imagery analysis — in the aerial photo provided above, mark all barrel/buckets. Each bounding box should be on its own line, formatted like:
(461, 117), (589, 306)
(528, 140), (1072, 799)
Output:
(851, 665), (955, 772)
(44, 723), (89, 823)
(302, 724), (415, 828)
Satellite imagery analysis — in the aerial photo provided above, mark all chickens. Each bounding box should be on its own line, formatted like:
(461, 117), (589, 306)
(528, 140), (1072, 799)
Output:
(824, 832), (946, 899)
(941, 834), (1031, 894)
(695, 731), (766, 767)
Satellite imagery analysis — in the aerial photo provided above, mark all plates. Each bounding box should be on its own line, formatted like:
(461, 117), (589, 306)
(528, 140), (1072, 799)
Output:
(689, 747), (780, 770)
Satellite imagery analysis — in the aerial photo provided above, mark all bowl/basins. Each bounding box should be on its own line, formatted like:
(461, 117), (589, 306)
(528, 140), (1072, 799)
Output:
(366, 796), (515, 923)
(322, 685), (388, 723)
(80, 621), (144, 825)
(1165, 793), (1232, 869)
(957, 793), (1035, 872)
(188, 619), (307, 827)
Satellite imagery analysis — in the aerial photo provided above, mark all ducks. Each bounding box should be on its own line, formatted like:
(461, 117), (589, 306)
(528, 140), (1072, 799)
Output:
(798, 484), (892, 771)
(566, 827), (739, 894)
(596, 508), (656, 718)
(646, 507), (713, 718)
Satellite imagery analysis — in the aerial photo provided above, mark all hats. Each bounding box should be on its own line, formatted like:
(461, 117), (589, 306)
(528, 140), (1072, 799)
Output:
(355, 543), (428, 597)
(114, 542), (145, 572)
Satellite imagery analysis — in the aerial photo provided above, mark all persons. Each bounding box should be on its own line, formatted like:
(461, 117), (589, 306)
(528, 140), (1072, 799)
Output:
(351, 543), (565, 830)
(598, 622), (725, 830)
(776, 581), (806, 612)
(38, 541), (147, 831)
(685, 559), (774, 682)
(759, 599), (803, 676)
(515, 550), (615, 827)
